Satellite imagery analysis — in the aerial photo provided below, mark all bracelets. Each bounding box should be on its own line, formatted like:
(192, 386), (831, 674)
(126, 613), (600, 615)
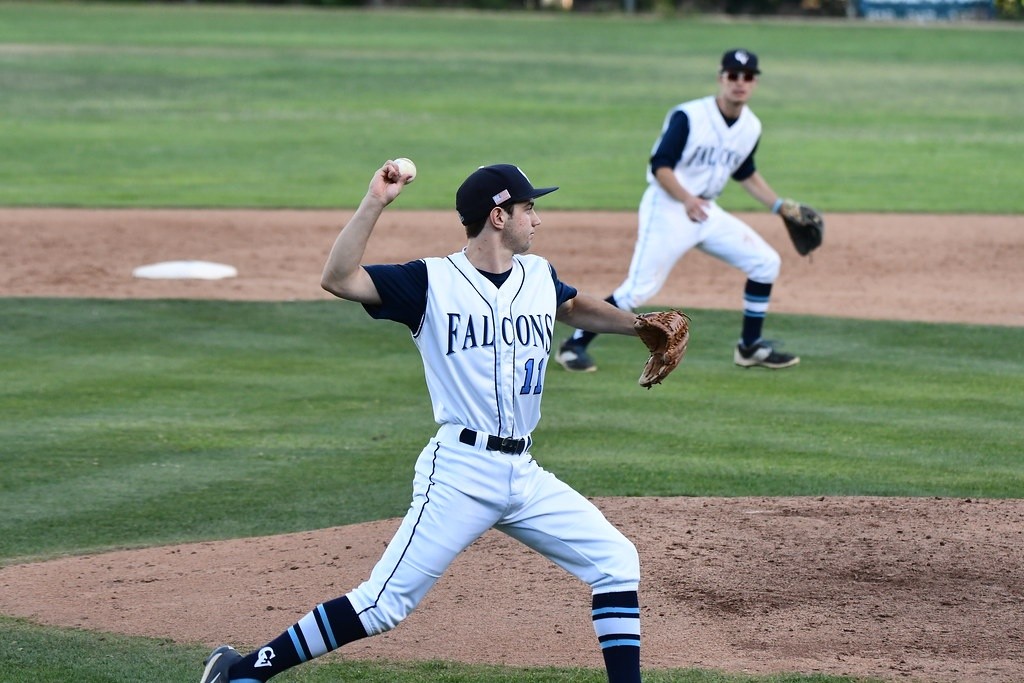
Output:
(772, 198), (783, 215)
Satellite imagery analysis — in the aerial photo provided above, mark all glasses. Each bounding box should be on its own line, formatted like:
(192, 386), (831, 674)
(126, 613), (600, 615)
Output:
(728, 71), (754, 81)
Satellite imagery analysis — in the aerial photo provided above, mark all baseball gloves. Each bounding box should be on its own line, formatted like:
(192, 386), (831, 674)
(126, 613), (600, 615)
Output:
(781, 204), (825, 257)
(634, 308), (692, 389)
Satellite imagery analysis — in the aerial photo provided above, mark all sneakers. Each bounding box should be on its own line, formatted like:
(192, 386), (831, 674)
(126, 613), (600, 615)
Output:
(734, 336), (800, 368)
(200, 645), (244, 683)
(554, 341), (597, 372)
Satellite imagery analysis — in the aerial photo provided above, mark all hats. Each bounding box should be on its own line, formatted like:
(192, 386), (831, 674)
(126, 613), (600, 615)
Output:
(722, 49), (760, 74)
(456, 164), (559, 227)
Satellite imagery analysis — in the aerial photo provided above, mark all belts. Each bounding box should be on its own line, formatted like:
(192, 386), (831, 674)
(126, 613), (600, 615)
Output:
(460, 428), (531, 455)
(698, 195), (705, 199)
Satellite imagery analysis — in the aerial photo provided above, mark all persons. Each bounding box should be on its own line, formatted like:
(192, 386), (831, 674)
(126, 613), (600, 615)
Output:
(555, 48), (822, 374)
(201, 153), (690, 683)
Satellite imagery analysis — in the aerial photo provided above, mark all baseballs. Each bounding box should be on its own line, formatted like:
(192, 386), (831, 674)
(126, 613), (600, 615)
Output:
(394, 157), (417, 183)
